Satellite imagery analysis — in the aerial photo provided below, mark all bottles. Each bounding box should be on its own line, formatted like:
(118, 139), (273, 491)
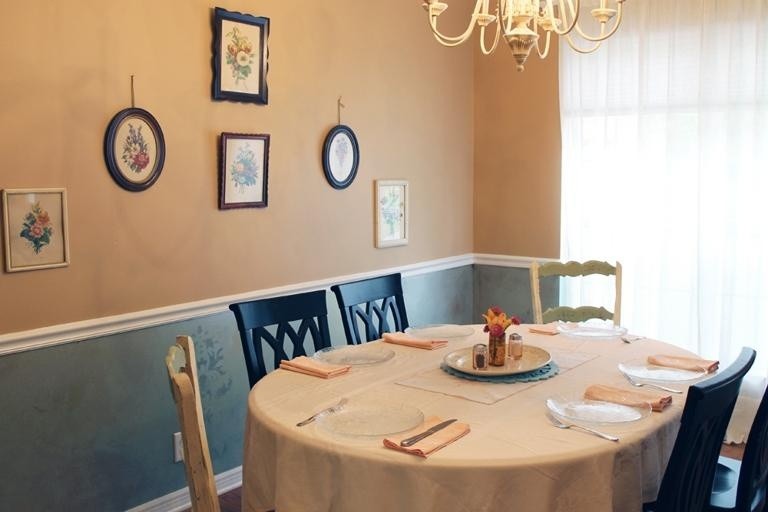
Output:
(508, 332), (524, 361)
(472, 343), (490, 372)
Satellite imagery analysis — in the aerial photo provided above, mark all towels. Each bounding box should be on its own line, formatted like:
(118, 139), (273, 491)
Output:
(382, 410), (471, 459)
(381, 325), (449, 361)
(585, 380), (673, 421)
(527, 326), (561, 336)
(279, 345), (354, 381)
(651, 351), (720, 379)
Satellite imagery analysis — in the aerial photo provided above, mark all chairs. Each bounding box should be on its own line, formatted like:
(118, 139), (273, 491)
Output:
(229, 289), (332, 397)
(169, 334), (236, 512)
(713, 383), (766, 511)
(331, 272), (410, 344)
(528, 262), (622, 327)
(648, 346), (757, 512)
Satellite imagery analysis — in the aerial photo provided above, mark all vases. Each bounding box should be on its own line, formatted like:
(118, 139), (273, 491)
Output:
(489, 340), (508, 369)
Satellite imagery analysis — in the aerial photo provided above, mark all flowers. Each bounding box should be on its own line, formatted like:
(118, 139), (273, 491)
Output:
(483, 311), (518, 340)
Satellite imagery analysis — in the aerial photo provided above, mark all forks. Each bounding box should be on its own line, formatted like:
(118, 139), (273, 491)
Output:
(551, 421), (620, 442)
(623, 373), (683, 395)
(296, 397), (348, 427)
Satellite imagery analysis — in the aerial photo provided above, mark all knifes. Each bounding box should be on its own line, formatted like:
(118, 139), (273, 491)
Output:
(401, 417), (458, 447)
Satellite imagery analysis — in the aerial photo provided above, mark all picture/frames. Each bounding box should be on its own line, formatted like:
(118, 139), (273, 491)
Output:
(211, 6), (271, 105)
(374, 179), (409, 249)
(220, 131), (269, 210)
(3, 188), (73, 272)
(321, 125), (361, 190)
(102, 75), (167, 192)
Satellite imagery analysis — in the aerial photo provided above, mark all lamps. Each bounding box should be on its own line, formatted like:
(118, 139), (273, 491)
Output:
(421, 0), (623, 73)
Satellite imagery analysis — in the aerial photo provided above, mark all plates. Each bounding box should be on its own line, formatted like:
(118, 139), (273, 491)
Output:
(618, 358), (708, 382)
(405, 323), (475, 340)
(322, 404), (424, 437)
(442, 342), (551, 376)
(313, 344), (396, 366)
(555, 322), (630, 340)
(544, 392), (652, 425)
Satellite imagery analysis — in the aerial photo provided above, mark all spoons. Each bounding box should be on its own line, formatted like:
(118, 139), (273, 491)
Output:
(620, 336), (646, 343)
